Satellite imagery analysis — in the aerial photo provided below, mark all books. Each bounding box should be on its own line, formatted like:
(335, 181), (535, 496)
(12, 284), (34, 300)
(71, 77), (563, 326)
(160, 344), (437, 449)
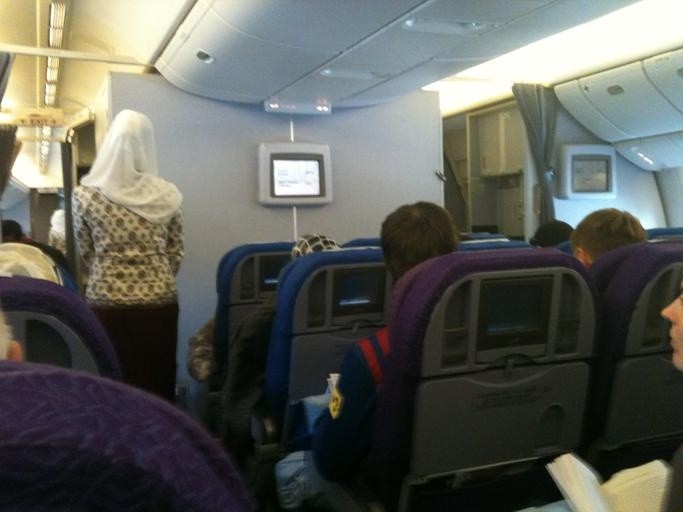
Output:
(543, 452), (671, 512)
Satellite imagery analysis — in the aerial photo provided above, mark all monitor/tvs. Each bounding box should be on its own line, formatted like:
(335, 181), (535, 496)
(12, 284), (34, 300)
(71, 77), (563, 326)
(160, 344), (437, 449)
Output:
(477, 274), (553, 350)
(332, 267), (386, 324)
(557, 143), (618, 200)
(257, 141), (333, 207)
(259, 254), (292, 297)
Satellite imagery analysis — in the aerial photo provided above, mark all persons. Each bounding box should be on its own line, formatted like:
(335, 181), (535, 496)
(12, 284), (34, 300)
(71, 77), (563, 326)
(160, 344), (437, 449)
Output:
(0, 219), (49, 256)
(50, 208), (67, 257)
(529, 219), (573, 250)
(274, 201), (457, 512)
(50, 109), (186, 408)
(217, 233), (342, 465)
(600, 279), (683, 512)
(569, 208), (647, 268)
(0, 310), (23, 363)
